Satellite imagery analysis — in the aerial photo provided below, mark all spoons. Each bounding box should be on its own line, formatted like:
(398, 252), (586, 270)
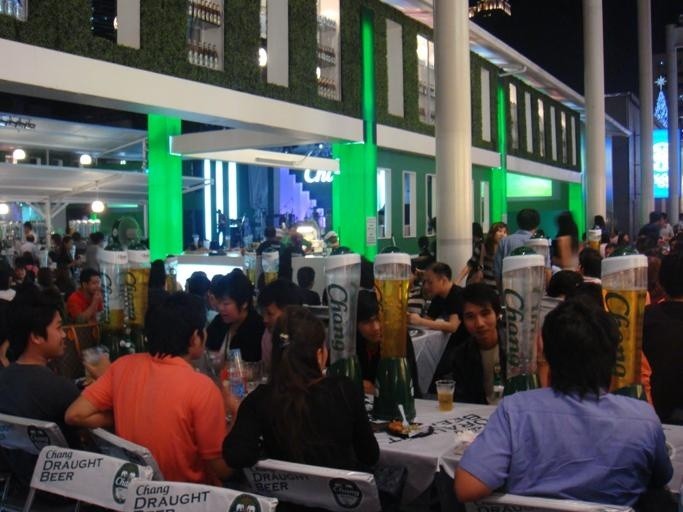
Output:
(398, 404), (410, 427)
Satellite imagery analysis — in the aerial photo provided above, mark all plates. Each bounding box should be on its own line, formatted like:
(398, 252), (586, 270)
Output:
(386, 421), (434, 440)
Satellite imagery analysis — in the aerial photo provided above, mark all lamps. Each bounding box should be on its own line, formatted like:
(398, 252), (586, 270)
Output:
(13, 148), (26, 160)
(469, 0), (512, 19)
(0, 204), (9, 214)
(91, 180), (105, 213)
(80, 153), (91, 165)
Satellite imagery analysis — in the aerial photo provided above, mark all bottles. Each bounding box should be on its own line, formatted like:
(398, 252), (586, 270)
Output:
(225, 348), (245, 422)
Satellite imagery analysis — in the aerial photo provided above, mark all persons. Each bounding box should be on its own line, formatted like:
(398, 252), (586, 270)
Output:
(0, 208), (682, 427)
(222, 306), (379, 512)
(454, 297), (674, 512)
(0, 291), (112, 512)
(64, 290), (245, 488)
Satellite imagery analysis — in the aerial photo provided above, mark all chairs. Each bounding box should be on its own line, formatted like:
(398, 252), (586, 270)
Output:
(465, 493), (632, 511)
(22, 446), (153, 512)
(0, 414), (81, 512)
(89, 427), (165, 482)
(243, 459), (381, 512)
(125, 478), (278, 512)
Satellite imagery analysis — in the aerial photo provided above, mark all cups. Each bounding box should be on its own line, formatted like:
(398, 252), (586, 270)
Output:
(81, 346), (103, 376)
(435, 379), (456, 412)
